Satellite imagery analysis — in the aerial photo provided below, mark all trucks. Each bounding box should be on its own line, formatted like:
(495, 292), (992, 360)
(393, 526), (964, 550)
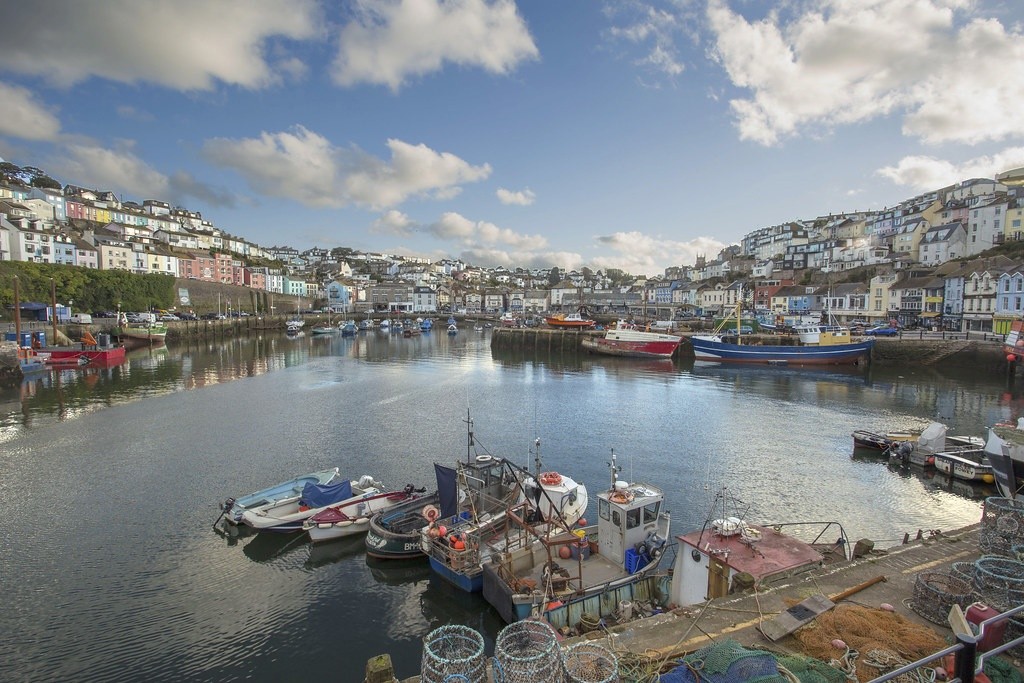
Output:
(71, 313), (92, 324)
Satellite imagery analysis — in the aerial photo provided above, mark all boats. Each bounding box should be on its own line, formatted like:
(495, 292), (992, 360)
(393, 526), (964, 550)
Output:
(364, 407), (532, 560)
(582, 318), (686, 359)
(302, 482), (429, 542)
(851, 420), (1024, 509)
(416, 436), (588, 594)
(219, 466), (340, 528)
(481, 446), (670, 636)
(239, 474), (384, 535)
(690, 283), (877, 365)
(544, 315), (597, 327)
(284, 292), (545, 337)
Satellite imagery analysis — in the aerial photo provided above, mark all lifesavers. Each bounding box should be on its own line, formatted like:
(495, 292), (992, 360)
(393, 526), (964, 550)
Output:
(541, 472), (562, 485)
(476, 455), (491, 461)
(994, 423), (1015, 428)
(610, 491), (634, 504)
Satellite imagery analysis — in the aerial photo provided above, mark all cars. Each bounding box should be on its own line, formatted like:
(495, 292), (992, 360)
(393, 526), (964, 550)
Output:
(851, 318), (897, 338)
(95, 308), (268, 323)
(363, 308), (494, 315)
(311, 306), (334, 314)
